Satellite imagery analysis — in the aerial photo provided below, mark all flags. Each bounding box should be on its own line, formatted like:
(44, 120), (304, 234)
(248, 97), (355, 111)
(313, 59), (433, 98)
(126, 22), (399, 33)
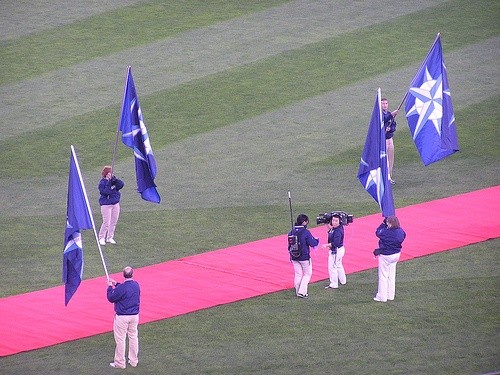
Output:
(119, 67), (161, 204)
(357, 89), (395, 217)
(63, 149), (94, 307)
(405, 34), (461, 168)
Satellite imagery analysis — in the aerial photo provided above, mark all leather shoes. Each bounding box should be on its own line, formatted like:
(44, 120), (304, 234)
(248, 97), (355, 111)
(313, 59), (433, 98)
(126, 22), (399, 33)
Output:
(110, 363), (118, 368)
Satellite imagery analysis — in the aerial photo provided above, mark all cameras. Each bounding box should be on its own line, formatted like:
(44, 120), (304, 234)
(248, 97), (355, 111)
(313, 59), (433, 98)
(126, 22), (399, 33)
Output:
(373, 249), (384, 255)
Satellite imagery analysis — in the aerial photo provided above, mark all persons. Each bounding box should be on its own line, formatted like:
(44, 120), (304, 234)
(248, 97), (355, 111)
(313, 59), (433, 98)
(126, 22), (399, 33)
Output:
(373, 215), (406, 302)
(98, 166), (125, 246)
(106, 266), (140, 369)
(325, 215), (347, 289)
(287, 214), (319, 298)
(381, 98), (398, 185)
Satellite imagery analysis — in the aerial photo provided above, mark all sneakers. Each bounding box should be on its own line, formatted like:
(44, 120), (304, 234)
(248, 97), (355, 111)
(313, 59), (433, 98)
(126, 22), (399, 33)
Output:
(99, 238), (106, 245)
(297, 293), (309, 298)
(374, 297), (387, 302)
(325, 284), (338, 289)
(389, 178), (395, 184)
(106, 238), (116, 244)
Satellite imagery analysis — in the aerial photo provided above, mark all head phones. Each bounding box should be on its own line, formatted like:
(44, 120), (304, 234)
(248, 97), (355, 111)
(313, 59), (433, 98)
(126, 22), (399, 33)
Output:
(330, 214), (342, 225)
